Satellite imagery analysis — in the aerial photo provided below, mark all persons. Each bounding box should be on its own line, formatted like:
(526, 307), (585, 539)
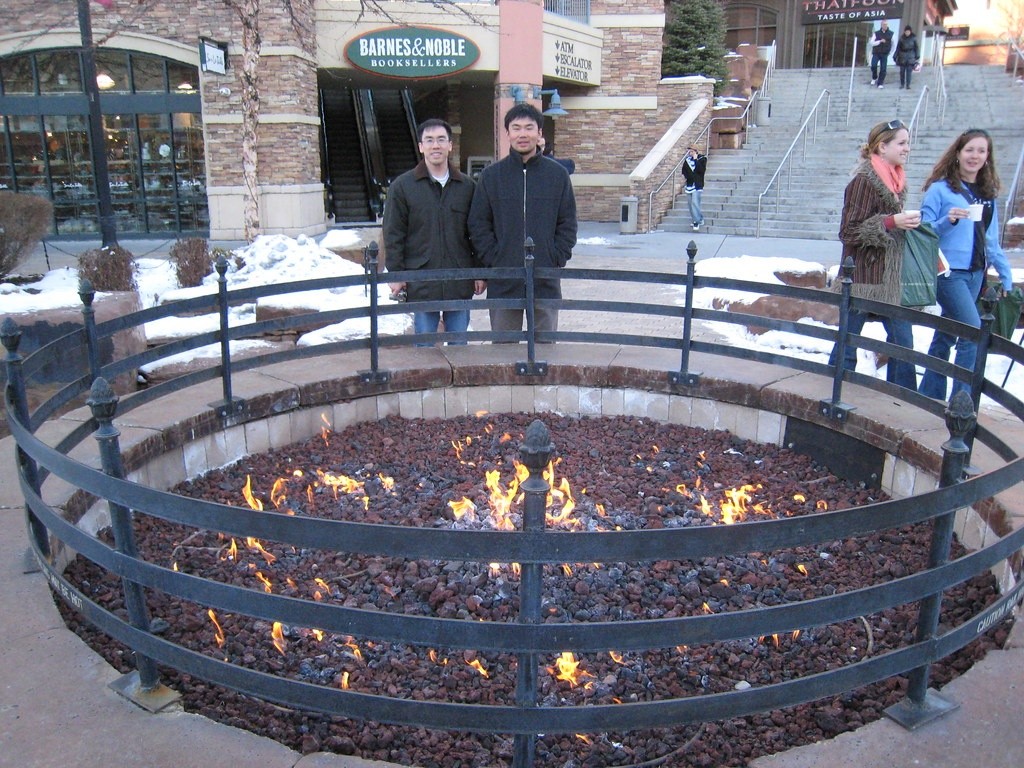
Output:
(382, 119), (489, 348)
(870, 20), (894, 88)
(893, 26), (920, 89)
(828, 120), (921, 392)
(467, 103), (578, 344)
(682, 143), (707, 231)
(920, 128), (1012, 403)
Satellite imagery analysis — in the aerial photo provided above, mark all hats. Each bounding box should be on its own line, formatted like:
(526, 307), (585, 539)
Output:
(904, 25), (912, 31)
(687, 144), (698, 150)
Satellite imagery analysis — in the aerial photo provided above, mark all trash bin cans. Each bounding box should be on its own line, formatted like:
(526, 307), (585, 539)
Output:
(619, 197), (638, 235)
(757, 97), (771, 126)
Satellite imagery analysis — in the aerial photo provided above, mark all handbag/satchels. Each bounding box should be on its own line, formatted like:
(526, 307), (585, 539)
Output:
(899, 221), (939, 307)
(912, 60), (920, 71)
(975, 283), (1024, 341)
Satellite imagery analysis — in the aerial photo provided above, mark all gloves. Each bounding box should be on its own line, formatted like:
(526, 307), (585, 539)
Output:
(894, 58), (896, 62)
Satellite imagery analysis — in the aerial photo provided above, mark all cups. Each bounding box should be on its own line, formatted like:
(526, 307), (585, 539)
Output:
(966, 205), (984, 221)
(905, 210), (920, 226)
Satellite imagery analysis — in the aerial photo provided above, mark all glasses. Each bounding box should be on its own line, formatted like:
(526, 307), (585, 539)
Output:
(872, 119), (903, 141)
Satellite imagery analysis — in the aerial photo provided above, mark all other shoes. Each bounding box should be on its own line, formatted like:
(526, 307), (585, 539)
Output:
(871, 78), (878, 85)
(877, 84), (884, 88)
(906, 86), (911, 89)
(693, 222), (699, 230)
(690, 220), (704, 226)
(899, 85), (904, 89)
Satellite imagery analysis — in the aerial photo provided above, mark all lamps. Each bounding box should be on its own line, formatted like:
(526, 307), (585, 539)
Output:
(96, 68), (116, 90)
(532, 85), (570, 120)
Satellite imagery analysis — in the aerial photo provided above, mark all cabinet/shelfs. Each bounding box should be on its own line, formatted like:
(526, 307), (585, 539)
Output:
(16, 184), (207, 226)
